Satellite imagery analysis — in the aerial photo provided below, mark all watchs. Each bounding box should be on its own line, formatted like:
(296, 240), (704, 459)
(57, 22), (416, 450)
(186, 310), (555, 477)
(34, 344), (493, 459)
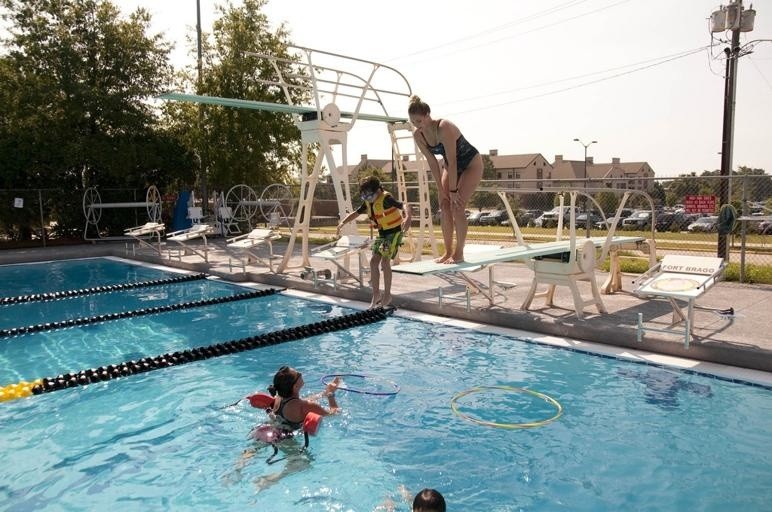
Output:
(450, 188), (458, 193)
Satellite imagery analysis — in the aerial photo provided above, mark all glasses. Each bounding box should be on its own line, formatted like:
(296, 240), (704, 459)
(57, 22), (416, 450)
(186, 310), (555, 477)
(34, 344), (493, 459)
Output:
(291, 372), (302, 389)
(360, 188), (377, 201)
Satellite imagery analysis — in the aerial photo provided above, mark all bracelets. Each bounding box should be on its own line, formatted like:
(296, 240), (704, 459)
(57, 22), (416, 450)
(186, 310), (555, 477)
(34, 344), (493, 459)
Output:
(327, 392), (335, 399)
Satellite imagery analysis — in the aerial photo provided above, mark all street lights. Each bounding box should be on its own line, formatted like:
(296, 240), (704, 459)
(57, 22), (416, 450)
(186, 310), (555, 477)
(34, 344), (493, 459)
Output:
(707, 0), (756, 262)
(573, 138), (598, 238)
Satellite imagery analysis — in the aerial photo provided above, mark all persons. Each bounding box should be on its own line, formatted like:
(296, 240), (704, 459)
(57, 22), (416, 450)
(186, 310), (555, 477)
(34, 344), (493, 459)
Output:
(219, 365), (341, 497)
(336, 174), (412, 309)
(408, 94), (484, 264)
(412, 488), (447, 511)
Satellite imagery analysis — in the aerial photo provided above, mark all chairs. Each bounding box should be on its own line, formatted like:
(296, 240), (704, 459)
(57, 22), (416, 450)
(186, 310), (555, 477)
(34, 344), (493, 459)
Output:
(630, 255), (734, 351)
(122, 222), (283, 276)
(310, 235), (373, 292)
